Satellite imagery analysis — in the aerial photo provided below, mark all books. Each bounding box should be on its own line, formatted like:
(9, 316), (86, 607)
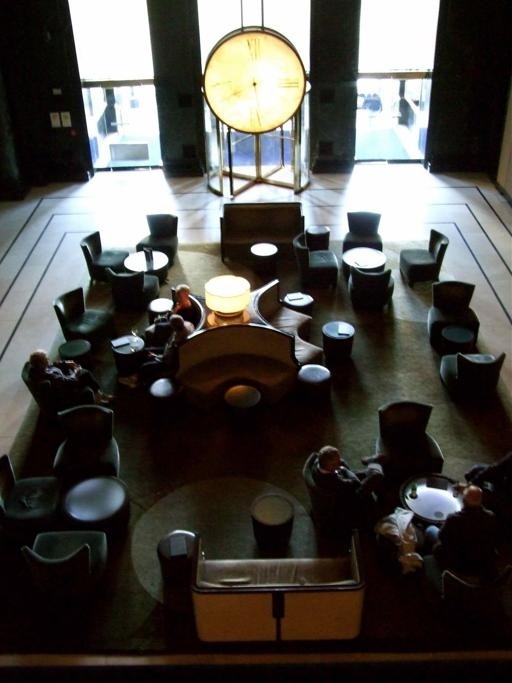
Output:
(287, 292), (303, 300)
(390, 508), (414, 532)
(110, 338), (131, 348)
(337, 322), (351, 335)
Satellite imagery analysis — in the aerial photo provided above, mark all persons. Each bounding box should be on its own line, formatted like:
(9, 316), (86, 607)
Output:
(428, 484), (503, 578)
(26, 352), (115, 412)
(310, 445), (403, 513)
(128, 314), (195, 389)
(140, 284), (194, 348)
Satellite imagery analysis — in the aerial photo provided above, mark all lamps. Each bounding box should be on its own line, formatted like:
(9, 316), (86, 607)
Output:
(204, 274), (252, 330)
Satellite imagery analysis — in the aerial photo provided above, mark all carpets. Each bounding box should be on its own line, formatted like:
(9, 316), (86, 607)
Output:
(7, 240), (512, 656)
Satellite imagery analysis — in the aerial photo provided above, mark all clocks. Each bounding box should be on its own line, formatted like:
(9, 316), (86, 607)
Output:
(202, 25), (307, 135)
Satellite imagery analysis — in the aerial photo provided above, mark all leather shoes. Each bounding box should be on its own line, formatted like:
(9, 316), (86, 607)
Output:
(101, 392), (117, 402)
(99, 399), (112, 407)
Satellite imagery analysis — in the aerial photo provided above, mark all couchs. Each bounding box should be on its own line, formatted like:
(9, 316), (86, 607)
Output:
(343, 212), (383, 254)
(191, 532), (367, 643)
(440, 352), (506, 393)
(105, 267), (160, 309)
(375, 399), (444, 474)
(20, 531), (108, 569)
(80, 231), (129, 282)
(302, 452), (319, 510)
(21, 361), (51, 409)
(253, 278), (325, 366)
(136, 214), (178, 265)
(399, 228), (449, 289)
(53, 287), (114, 343)
(293, 233), (338, 290)
(52, 404), (120, 478)
(0, 453), (61, 525)
(178, 322), (299, 398)
(220, 202), (304, 263)
(348, 266), (394, 314)
(428, 280), (480, 344)
(170, 287), (207, 333)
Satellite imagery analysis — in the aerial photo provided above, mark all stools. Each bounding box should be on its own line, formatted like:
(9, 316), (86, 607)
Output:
(59, 339), (91, 364)
(157, 529), (200, 577)
(298, 364), (332, 404)
(150, 378), (176, 397)
(249, 493), (295, 547)
(306, 226), (330, 252)
(442, 326), (474, 343)
